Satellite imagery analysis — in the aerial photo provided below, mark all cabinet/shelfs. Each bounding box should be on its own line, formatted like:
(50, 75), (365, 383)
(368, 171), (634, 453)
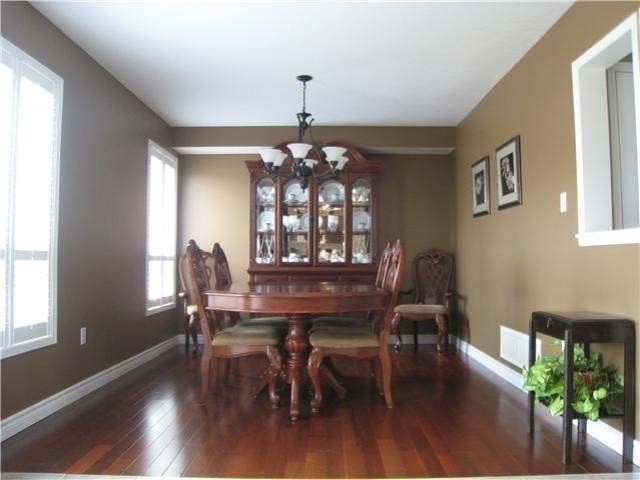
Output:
(244, 139), (383, 283)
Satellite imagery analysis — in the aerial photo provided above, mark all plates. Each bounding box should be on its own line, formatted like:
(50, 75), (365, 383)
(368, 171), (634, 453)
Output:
(257, 180), (372, 233)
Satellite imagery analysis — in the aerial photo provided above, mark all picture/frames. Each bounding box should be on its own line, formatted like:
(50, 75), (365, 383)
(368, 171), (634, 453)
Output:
(471, 134), (522, 218)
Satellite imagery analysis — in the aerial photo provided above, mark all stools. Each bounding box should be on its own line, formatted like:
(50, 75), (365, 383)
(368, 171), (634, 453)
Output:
(529, 310), (635, 472)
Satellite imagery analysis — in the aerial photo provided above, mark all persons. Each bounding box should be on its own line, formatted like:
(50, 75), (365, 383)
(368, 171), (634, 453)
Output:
(500, 157), (514, 195)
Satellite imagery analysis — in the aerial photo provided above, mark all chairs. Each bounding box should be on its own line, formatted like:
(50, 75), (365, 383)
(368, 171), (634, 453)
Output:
(392, 248), (453, 351)
(210, 242), (290, 381)
(181, 240), (284, 410)
(306, 240), (405, 414)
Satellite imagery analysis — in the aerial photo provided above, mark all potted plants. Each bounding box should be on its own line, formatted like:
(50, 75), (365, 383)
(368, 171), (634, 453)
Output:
(522, 337), (624, 421)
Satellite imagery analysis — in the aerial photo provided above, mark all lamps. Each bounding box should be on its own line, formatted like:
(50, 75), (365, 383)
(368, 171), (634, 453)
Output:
(257, 75), (350, 195)
